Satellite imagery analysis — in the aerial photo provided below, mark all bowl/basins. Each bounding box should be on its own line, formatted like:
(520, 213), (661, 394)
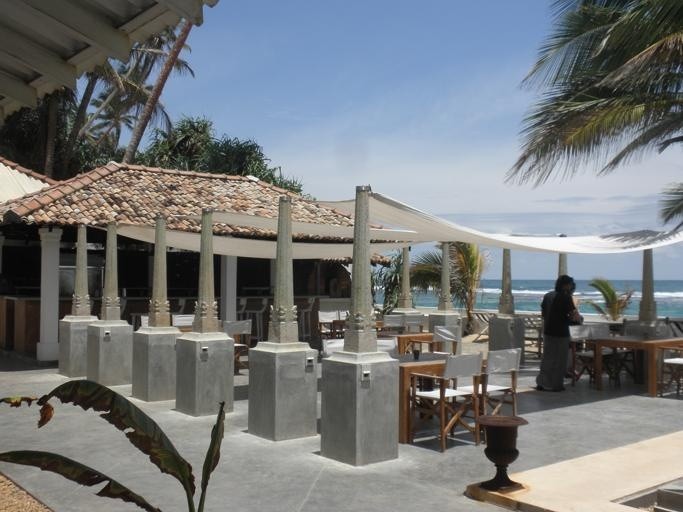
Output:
(568, 323), (612, 338)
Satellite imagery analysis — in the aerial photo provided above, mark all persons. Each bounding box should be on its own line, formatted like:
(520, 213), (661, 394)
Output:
(564, 283), (581, 378)
(534, 274), (584, 392)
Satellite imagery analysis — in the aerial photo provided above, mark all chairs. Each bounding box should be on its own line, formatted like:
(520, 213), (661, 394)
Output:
(523, 312), (682, 398)
(169, 315), (253, 376)
(321, 311), (522, 453)
